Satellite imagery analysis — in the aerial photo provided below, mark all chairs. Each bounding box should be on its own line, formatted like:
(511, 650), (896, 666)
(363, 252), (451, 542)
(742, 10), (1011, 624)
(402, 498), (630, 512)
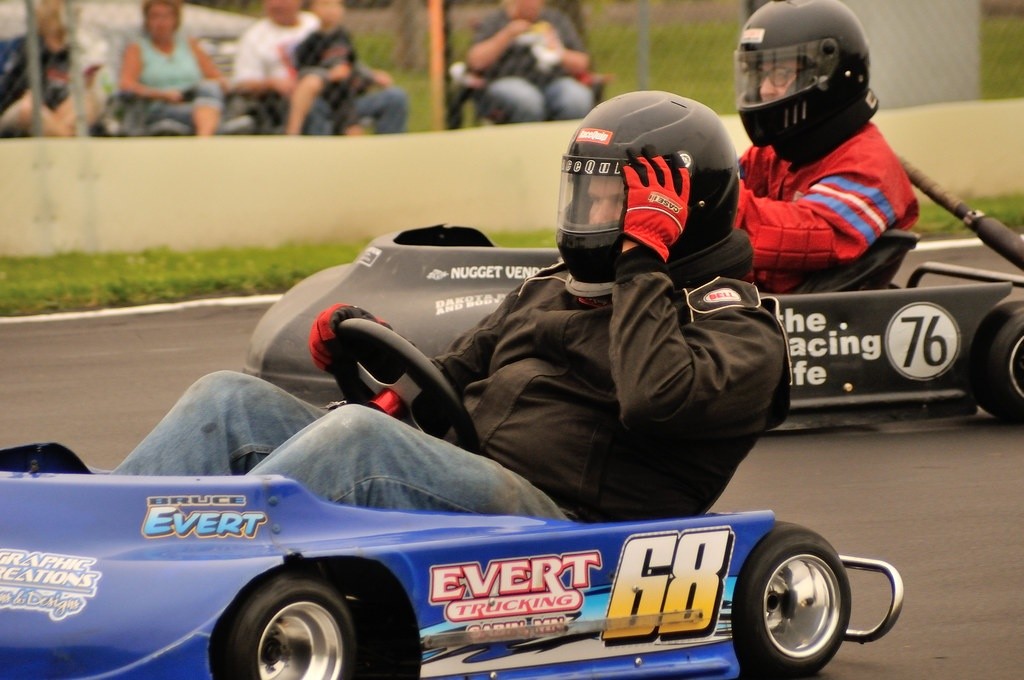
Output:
(98, 23), (620, 135)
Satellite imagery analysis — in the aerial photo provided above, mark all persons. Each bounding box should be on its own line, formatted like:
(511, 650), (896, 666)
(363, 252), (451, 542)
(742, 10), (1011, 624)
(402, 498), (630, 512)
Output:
(0, 0), (115, 136)
(120, 0), (252, 136)
(467, 0), (595, 123)
(732, 0), (919, 295)
(233, 0), (409, 135)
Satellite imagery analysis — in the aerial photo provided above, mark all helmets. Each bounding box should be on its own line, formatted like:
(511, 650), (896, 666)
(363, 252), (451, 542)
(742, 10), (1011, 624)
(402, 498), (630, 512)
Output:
(556, 90), (739, 295)
(734, 1), (870, 147)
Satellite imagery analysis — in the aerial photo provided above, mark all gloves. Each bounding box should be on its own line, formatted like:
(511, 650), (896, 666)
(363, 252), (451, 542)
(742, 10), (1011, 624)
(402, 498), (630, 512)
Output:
(610, 143), (690, 264)
(308, 304), (395, 373)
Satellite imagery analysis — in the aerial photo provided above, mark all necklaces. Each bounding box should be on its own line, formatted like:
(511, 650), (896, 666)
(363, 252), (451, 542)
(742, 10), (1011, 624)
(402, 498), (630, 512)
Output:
(110, 90), (791, 520)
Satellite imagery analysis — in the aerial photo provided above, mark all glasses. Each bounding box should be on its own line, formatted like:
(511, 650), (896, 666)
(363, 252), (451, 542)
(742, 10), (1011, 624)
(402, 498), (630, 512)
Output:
(744, 65), (815, 87)
